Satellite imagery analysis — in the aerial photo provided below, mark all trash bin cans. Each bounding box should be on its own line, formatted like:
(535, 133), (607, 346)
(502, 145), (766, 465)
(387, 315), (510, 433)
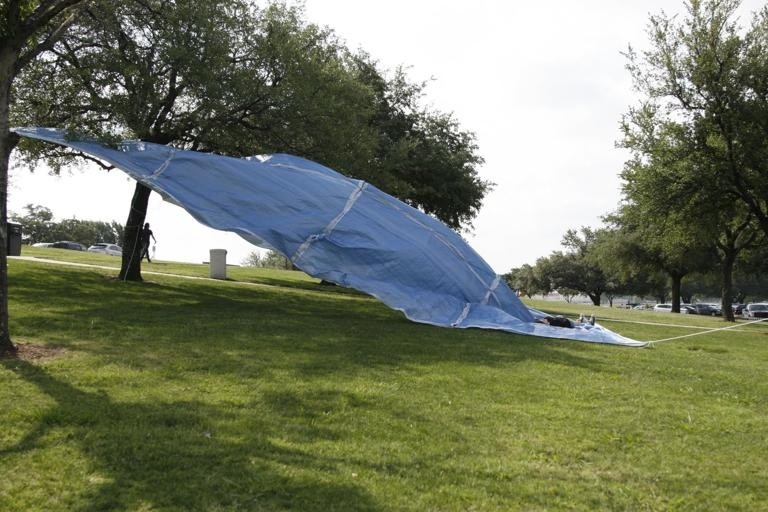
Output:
(211, 249), (227, 280)
(7, 222), (23, 256)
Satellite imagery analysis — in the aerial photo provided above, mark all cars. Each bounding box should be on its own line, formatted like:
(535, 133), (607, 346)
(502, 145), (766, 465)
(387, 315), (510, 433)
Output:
(30, 242), (53, 249)
(54, 241), (86, 251)
(88, 243), (122, 257)
(626, 302), (767, 318)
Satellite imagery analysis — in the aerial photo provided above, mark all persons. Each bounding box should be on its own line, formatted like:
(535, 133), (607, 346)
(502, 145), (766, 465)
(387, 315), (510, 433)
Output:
(139, 222), (158, 264)
(534, 312), (595, 329)
